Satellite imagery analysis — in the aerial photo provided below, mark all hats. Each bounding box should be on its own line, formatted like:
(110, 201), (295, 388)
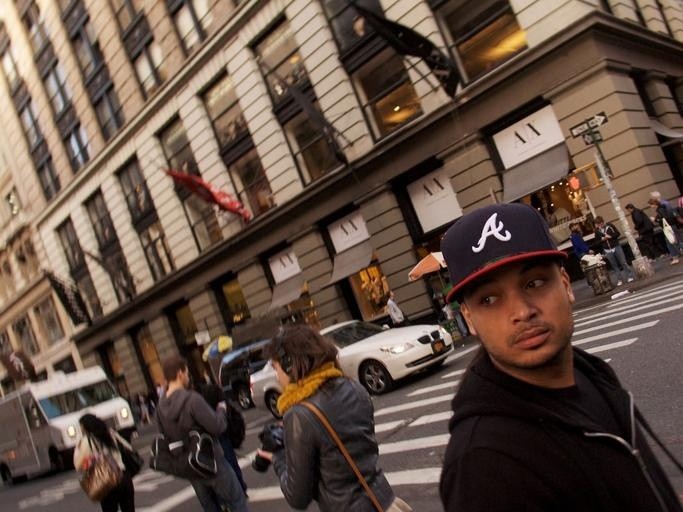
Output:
(439, 204), (569, 304)
(569, 222), (578, 229)
(649, 191), (661, 203)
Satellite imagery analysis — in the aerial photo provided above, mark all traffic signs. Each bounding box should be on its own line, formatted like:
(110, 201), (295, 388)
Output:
(568, 110), (609, 145)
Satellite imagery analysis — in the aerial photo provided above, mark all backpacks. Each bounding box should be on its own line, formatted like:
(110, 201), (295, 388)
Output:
(227, 406), (245, 448)
(672, 207), (683, 230)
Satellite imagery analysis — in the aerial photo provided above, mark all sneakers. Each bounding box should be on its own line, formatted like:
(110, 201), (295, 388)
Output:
(669, 259), (679, 266)
(616, 280), (623, 286)
(627, 277), (634, 282)
(656, 252), (668, 260)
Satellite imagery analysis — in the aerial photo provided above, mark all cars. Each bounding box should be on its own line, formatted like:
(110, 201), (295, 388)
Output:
(217, 319), (455, 420)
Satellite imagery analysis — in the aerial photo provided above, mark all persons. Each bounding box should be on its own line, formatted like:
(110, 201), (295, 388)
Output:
(646, 198), (683, 266)
(439, 200), (682, 512)
(385, 289), (411, 327)
(190, 361), (250, 499)
(567, 222), (594, 262)
(591, 213), (635, 289)
(154, 357), (258, 511)
(625, 203), (669, 264)
(255, 322), (414, 512)
(649, 191), (679, 230)
(71, 413), (142, 511)
(121, 367), (218, 427)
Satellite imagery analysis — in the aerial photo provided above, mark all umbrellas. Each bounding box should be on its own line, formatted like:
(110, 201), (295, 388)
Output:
(202, 335), (233, 361)
(407, 251), (454, 290)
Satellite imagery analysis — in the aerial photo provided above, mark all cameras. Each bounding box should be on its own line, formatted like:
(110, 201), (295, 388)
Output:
(251, 423), (284, 472)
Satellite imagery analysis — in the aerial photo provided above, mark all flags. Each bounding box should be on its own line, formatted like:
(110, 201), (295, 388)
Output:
(82, 252), (136, 309)
(255, 56), (341, 158)
(0, 350), (37, 386)
(345, 0), (464, 102)
(149, 163), (252, 226)
(40, 266), (90, 329)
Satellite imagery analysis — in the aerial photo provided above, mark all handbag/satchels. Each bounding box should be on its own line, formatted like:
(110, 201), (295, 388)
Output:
(148, 431), (217, 482)
(579, 253), (607, 267)
(662, 218), (678, 245)
(78, 436), (124, 502)
(121, 450), (144, 478)
(606, 222), (621, 240)
(383, 496), (412, 512)
(638, 224), (652, 234)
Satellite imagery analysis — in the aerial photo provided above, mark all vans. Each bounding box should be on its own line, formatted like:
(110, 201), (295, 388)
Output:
(0, 364), (137, 486)
